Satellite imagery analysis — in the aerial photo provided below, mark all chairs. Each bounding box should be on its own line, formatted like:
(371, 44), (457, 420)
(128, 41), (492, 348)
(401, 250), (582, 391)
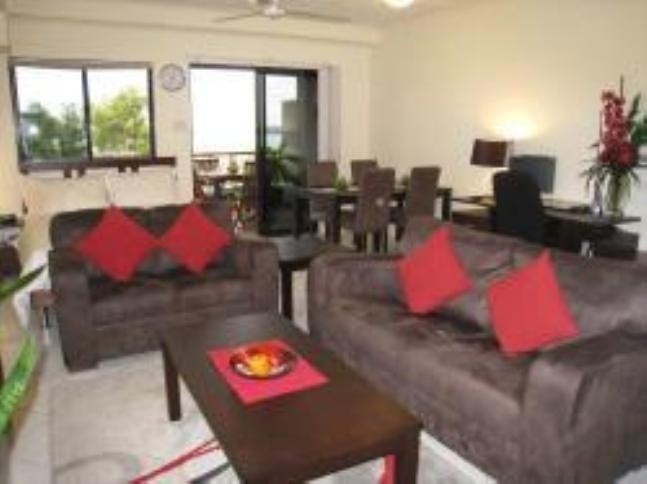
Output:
(492, 167), (557, 246)
(193, 159), (440, 253)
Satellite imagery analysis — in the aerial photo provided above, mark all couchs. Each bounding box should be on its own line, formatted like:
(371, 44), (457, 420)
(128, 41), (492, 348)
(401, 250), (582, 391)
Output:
(308, 215), (647, 483)
(48, 204), (280, 373)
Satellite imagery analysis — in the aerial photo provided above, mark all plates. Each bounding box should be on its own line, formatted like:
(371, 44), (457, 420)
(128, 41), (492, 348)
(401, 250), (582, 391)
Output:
(230, 349), (295, 378)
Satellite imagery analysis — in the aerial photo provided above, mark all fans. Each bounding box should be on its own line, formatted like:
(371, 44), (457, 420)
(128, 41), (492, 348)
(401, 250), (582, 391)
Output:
(210, 0), (352, 26)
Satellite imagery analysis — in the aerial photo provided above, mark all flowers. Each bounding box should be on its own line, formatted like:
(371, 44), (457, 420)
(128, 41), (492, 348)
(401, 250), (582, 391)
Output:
(579, 75), (647, 206)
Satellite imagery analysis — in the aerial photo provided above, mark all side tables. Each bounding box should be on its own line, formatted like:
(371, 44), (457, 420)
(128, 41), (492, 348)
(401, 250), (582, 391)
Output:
(266, 233), (354, 320)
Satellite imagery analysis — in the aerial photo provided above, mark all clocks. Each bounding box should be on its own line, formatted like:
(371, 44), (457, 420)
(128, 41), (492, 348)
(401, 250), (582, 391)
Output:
(157, 63), (185, 92)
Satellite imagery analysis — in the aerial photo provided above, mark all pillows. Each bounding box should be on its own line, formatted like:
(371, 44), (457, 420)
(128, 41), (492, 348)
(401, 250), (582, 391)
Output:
(395, 223), (579, 357)
(72, 203), (231, 284)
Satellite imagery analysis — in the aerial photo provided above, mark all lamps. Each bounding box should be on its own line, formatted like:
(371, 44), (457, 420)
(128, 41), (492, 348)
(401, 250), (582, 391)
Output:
(470, 139), (507, 198)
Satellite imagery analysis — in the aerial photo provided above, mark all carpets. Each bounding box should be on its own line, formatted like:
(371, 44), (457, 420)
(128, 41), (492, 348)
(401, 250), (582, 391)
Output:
(48, 363), (469, 484)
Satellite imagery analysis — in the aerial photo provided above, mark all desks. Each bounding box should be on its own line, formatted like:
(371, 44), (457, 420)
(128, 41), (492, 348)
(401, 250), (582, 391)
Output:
(458, 190), (643, 257)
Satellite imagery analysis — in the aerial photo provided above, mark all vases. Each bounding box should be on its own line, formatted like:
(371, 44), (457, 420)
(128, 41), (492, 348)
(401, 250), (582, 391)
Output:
(604, 174), (630, 211)
